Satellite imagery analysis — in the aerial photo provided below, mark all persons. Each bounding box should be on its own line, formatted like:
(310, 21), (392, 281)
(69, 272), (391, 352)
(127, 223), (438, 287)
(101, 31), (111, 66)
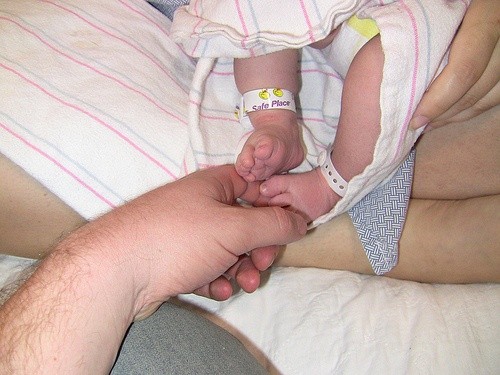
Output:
(0, 144), (304, 375)
(170, 0), (469, 230)
(274, 1), (500, 285)
